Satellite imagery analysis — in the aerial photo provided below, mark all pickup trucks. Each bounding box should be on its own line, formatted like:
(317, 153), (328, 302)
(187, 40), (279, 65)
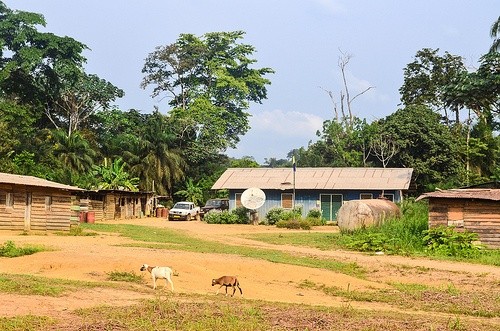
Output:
(168, 201), (200, 222)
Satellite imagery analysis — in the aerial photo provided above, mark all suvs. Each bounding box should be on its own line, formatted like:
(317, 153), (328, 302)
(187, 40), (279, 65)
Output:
(200, 199), (229, 221)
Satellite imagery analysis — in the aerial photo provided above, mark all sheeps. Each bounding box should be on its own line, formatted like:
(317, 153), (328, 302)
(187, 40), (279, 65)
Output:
(140, 264), (174, 292)
(211, 276), (242, 297)
